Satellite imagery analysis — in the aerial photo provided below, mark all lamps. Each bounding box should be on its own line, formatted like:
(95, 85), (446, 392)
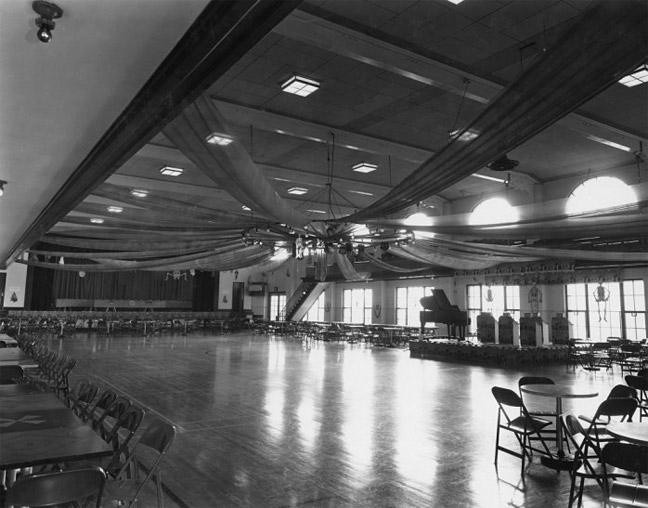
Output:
(450, 129), (478, 143)
(617, 64), (648, 89)
(243, 130), (415, 253)
(0, 180), (8, 197)
